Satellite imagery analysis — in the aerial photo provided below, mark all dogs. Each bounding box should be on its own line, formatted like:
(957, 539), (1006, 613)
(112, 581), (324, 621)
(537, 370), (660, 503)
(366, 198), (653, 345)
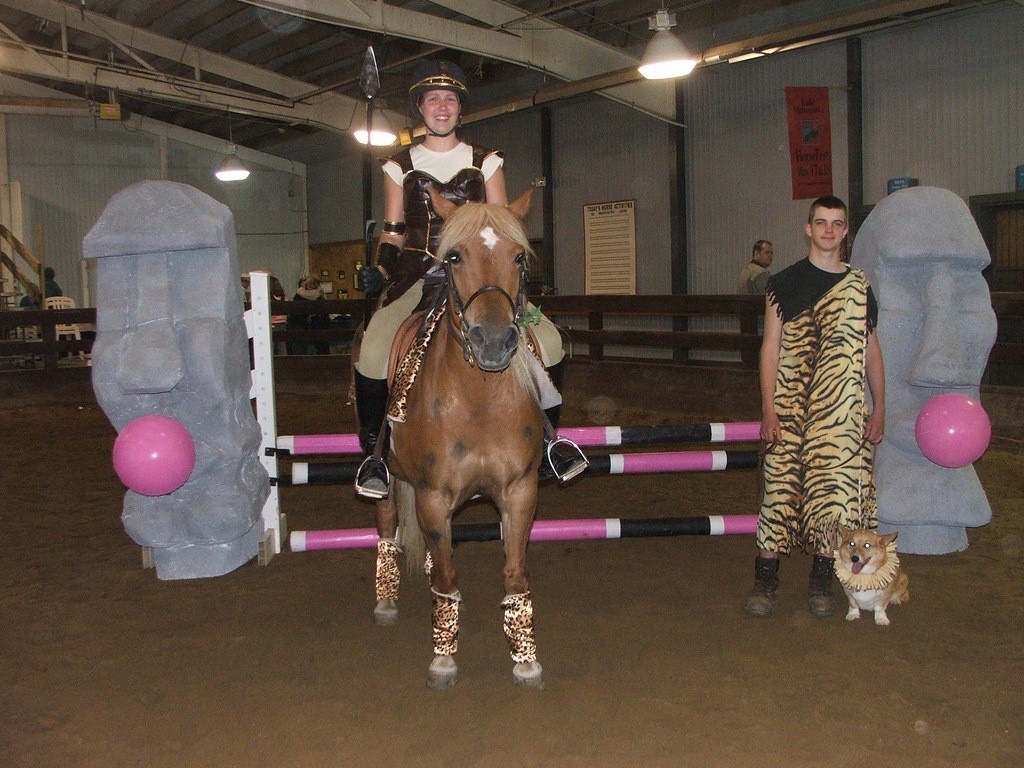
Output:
(835, 522), (910, 626)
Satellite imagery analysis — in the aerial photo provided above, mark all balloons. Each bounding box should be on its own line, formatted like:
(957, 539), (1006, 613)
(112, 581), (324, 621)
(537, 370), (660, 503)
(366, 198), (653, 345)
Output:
(112, 414), (196, 496)
(913, 394), (991, 468)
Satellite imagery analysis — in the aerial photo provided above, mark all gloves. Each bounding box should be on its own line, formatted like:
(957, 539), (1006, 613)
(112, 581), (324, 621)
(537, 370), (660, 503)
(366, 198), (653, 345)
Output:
(358, 266), (384, 293)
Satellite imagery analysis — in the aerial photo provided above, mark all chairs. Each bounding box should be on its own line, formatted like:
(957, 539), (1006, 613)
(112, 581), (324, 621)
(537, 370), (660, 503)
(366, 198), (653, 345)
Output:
(45, 296), (85, 361)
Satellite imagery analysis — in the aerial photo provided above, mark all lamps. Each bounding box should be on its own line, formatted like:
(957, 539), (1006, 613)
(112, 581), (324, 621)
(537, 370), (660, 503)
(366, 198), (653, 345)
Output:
(214, 111), (250, 182)
(354, 98), (397, 146)
(638, 1), (697, 79)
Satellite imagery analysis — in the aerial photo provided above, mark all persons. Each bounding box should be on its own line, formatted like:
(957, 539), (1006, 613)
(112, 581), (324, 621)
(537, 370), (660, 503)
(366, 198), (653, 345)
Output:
(354, 57), (584, 501)
(746, 195), (886, 618)
(742, 239), (774, 337)
(241, 268), (332, 355)
(20, 266), (63, 309)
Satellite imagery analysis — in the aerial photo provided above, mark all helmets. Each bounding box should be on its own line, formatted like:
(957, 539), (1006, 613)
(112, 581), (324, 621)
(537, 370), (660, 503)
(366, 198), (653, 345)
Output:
(409, 60), (472, 121)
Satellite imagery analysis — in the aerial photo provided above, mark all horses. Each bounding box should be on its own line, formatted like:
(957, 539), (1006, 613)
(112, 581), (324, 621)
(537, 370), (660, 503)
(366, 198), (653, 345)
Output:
(375, 188), (549, 689)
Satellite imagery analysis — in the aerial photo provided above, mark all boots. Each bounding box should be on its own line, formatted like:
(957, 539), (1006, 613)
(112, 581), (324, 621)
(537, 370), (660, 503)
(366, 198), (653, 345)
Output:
(746, 555), (781, 618)
(808, 555), (841, 618)
(538, 354), (583, 480)
(354, 365), (391, 504)
